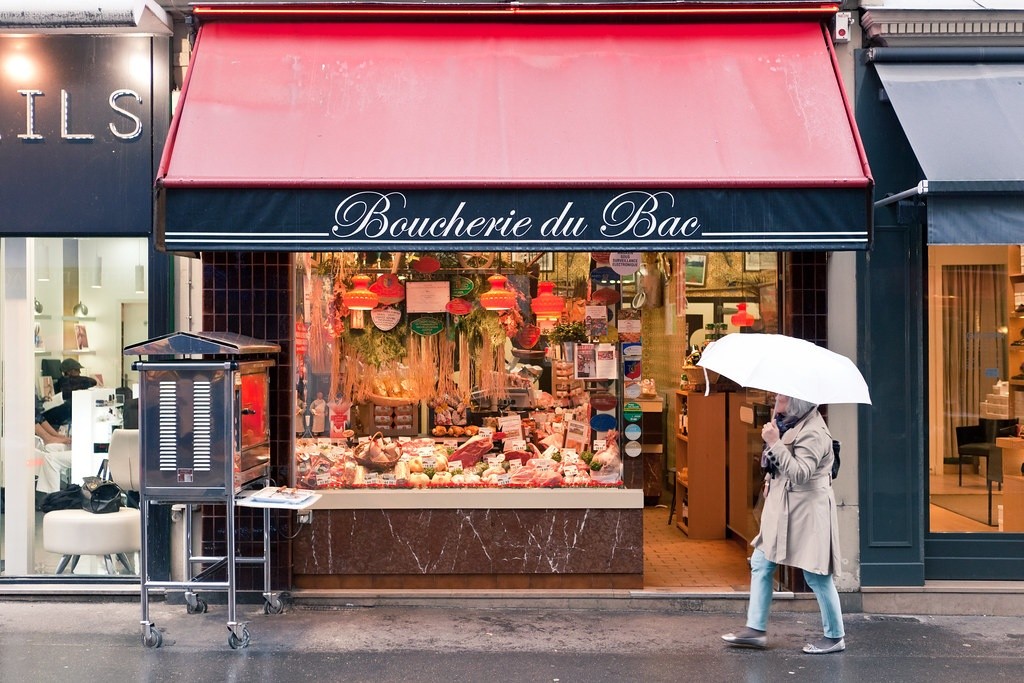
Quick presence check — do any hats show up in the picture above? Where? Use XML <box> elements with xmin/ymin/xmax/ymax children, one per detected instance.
<box><xmin>60</xmin><ymin>358</ymin><xmax>84</xmax><ymax>371</ymax></box>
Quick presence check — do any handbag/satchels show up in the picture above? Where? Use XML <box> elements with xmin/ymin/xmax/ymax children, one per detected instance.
<box><xmin>831</xmin><ymin>439</ymin><xmax>840</xmax><ymax>479</ymax></box>
<box><xmin>82</xmin><ymin>478</ymin><xmax>122</xmax><ymax>513</ymax></box>
<box><xmin>39</xmin><ymin>483</ymin><xmax>84</xmax><ymax>513</ymax></box>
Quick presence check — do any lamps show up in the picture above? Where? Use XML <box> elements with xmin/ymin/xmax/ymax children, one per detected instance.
<box><xmin>532</xmin><ymin>253</ymin><xmax>566</xmax><ymax>317</ymax></box>
<box><xmin>481</xmin><ymin>252</ymin><xmax>515</xmax><ymax>310</ymax></box>
<box><xmin>346</xmin><ymin>252</ymin><xmax>378</xmax><ymax>310</ymax></box>
<box><xmin>730</xmin><ymin>251</ymin><xmax>755</xmax><ymax>327</ymax></box>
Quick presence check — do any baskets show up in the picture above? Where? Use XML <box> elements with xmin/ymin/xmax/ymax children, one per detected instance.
<box><xmin>354</xmin><ymin>431</ymin><xmax>403</xmax><ymax>470</ymax></box>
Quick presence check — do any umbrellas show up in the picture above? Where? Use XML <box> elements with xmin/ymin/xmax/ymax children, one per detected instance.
<box><xmin>696</xmin><ymin>333</ymin><xmax>873</xmax><ymax>423</ymax></box>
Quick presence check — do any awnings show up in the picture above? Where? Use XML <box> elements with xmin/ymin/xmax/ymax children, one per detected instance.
<box><xmin>854</xmin><ymin>38</ymin><xmax>1024</xmax><ymax>244</ymax></box>
<box><xmin>150</xmin><ymin>14</ymin><xmax>875</xmax><ymax>254</ymax></box>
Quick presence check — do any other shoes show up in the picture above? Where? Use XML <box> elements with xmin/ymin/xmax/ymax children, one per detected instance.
<box><xmin>802</xmin><ymin>637</ymin><xmax>846</xmax><ymax>653</ymax></box>
<box><xmin>722</xmin><ymin>632</ymin><xmax>768</xmax><ymax>648</ymax></box>
<box><xmin>36</xmin><ymin>494</ymin><xmax>49</xmax><ymax>511</ymax></box>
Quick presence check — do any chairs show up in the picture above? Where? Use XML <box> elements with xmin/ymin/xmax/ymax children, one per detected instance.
<box><xmin>42</xmin><ymin>429</ymin><xmax>142</xmax><ymax>575</ymax></box>
<box><xmin>954</xmin><ymin>418</ymin><xmax>1019</xmax><ymax>485</ymax></box>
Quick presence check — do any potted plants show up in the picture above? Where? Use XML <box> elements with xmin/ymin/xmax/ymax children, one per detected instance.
<box><xmin>549</xmin><ymin>321</ymin><xmax>589</xmax><ymax>362</ymax></box>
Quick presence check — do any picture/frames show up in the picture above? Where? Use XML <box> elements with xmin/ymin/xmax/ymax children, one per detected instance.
<box><xmin>685</xmin><ymin>253</ymin><xmax>707</xmax><ymax>286</ymax></box>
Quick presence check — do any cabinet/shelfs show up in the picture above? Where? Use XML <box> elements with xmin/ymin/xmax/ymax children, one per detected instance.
<box><xmin>36</xmin><ymin>312</ymin><xmax>96</xmax><ymax>356</ymax></box>
<box><xmin>1007</xmin><ymin>314</ymin><xmax>1024</xmax><ymax>385</ymax></box>
<box><xmin>674</xmin><ymin>389</ymin><xmax>726</xmax><ymax>540</ymax></box>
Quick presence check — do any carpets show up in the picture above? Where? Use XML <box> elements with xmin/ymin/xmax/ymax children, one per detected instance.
<box><xmin>929</xmin><ymin>494</ymin><xmax>1003</xmax><ymax>526</ymax></box>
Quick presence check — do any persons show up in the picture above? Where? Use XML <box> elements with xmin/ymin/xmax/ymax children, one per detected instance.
<box><xmin>34</xmin><ymin>357</ymin><xmax>97</xmax><ymax>511</ymax></box>
<box><xmin>308</xmin><ymin>392</ymin><xmax>328</xmax><ymax>438</ymax></box>
<box><xmin>296</xmin><ymin>391</ymin><xmax>307</xmax><ymax>437</ymax></box>
<box><xmin>721</xmin><ymin>394</ymin><xmax>846</xmax><ymax>654</ymax></box>
